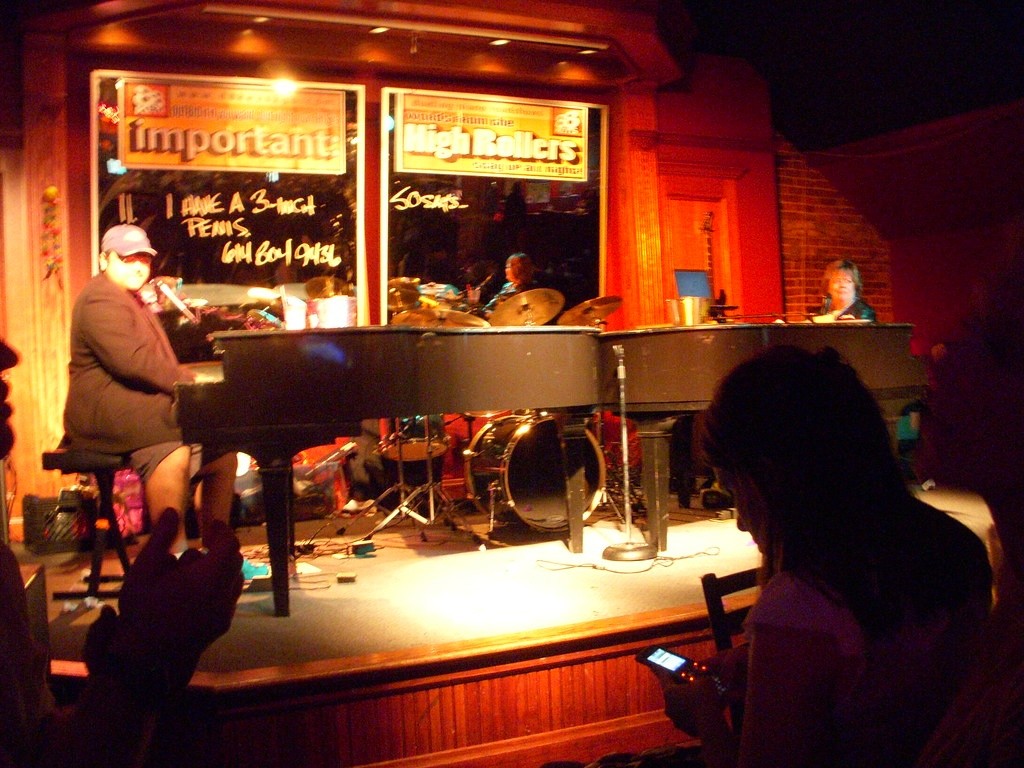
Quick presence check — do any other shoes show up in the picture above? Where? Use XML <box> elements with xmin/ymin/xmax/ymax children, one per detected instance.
<box><xmin>243</xmin><ymin>561</ymin><xmax>268</xmax><ymax>580</ymax></box>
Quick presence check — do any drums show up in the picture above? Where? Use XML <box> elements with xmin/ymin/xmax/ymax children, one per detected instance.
<box><xmin>377</xmin><ymin>439</ymin><xmax>447</xmax><ymax>507</ymax></box>
<box><xmin>388</xmin><ymin>277</ymin><xmax>421</xmax><ymax>308</ymax></box>
<box><xmin>463</xmin><ymin>415</ymin><xmax>606</xmax><ymax>532</ymax></box>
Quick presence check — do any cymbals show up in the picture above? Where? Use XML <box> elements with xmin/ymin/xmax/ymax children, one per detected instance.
<box><xmin>390</xmin><ymin>308</ymin><xmax>491</xmax><ymax>327</ymax></box>
<box><xmin>557</xmin><ymin>296</ymin><xmax>623</xmax><ymax>327</ymax></box>
<box><xmin>491</xmin><ymin>288</ymin><xmax>564</xmax><ymax>326</ymax></box>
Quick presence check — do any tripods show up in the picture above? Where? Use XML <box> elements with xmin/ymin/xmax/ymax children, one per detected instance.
<box><xmin>337</xmin><ymin>415</ymin><xmax>481</xmax><ymax>552</ymax></box>
<box><xmin>597</xmin><ymin>412</ymin><xmax>646</xmax><ymax>524</ymax></box>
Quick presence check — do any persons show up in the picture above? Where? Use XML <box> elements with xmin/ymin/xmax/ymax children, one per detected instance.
<box><xmin>1</xmin><ymin>335</ymin><xmax>246</xmax><ymax>768</ymax></box>
<box><xmin>63</xmin><ymin>222</ymin><xmax>273</xmax><ymax>579</ymax></box>
<box><xmin>646</xmin><ymin>340</ymin><xmax>994</xmax><ymax>768</ymax></box>
<box><xmin>906</xmin><ymin>242</ymin><xmax>1024</xmax><ymax>768</ymax></box>
<box><xmin>481</xmin><ymin>250</ymin><xmax>546</xmax><ymax>316</ymax></box>
<box><xmin>817</xmin><ymin>259</ymin><xmax>879</xmax><ymax>324</ymax></box>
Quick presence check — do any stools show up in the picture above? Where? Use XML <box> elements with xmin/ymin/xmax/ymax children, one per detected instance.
<box><xmin>42</xmin><ymin>451</ymin><xmax>132</xmax><ymax>600</ymax></box>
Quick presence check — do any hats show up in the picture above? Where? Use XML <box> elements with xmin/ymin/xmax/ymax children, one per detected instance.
<box><xmin>102</xmin><ymin>223</ymin><xmax>157</xmax><ymax>256</ymax></box>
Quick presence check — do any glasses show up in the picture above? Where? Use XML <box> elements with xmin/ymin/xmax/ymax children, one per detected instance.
<box><xmin>121</xmin><ymin>256</ymin><xmax>152</xmax><ymax>264</ymax></box>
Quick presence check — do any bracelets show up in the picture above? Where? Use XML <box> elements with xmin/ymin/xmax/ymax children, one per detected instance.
<box><xmin>81</xmin><ymin>605</ymin><xmax>204</xmax><ymax>706</ymax></box>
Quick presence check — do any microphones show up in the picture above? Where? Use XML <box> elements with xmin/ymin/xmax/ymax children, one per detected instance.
<box><xmin>821</xmin><ymin>293</ymin><xmax>832</xmax><ymax>315</ymax></box>
<box><xmin>157</xmin><ymin>281</ymin><xmax>198</xmax><ymax>324</ymax></box>
<box><xmin>475</xmin><ymin>273</ymin><xmax>496</xmax><ymax>291</ymax></box>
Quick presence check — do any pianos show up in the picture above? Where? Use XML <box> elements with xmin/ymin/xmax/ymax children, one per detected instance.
<box><xmin>598</xmin><ymin>321</ymin><xmax>930</xmax><ymax>553</ymax></box>
<box><xmin>173</xmin><ymin>327</ymin><xmax>604</xmax><ymax>617</ymax></box>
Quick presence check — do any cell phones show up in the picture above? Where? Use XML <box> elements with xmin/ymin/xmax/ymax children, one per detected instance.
<box><xmin>634</xmin><ymin>644</ymin><xmax>731</xmax><ymax>696</ymax></box>
